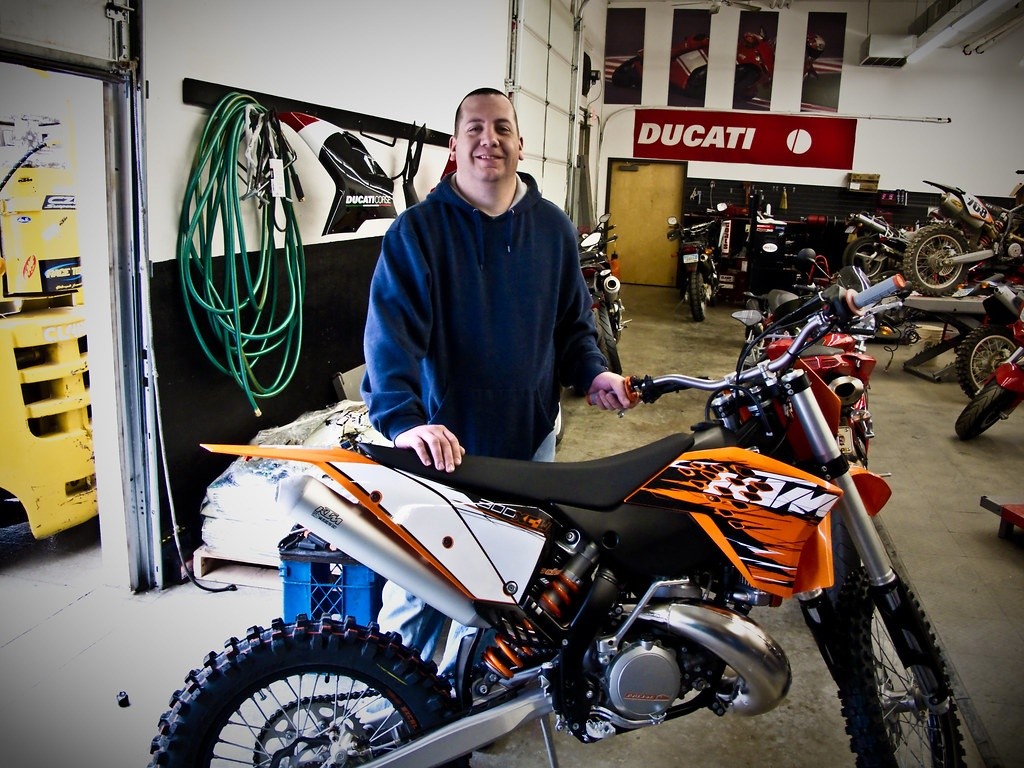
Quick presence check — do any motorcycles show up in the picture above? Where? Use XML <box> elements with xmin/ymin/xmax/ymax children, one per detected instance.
<box><xmin>841</xmin><ymin>209</ymin><xmax>906</xmax><ymax>282</ymax></box>
<box><xmin>577</xmin><ymin>212</ymin><xmax>634</xmax><ymax>383</ymax></box>
<box><xmin>147</xmin><ymin>272</ymin><xmax>966</xmax><ymax>768</ymax></box>
<box><xmin>903</xmin><ymin>169</ymin><xmax>1024</xmax><ymax>298</ymax></box>
<box><xmin>667</xmin><ymin>202</ymin><xmax>728</xmax><ymax>322</ymax></box>
<box><xmin>952</xmin><ymin>285</ymin><xmax>1023</xmax><ymax>443</ymax></box>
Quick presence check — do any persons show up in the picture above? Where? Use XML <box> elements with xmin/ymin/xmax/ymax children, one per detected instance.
<box><xmin>359</xmin><ymin>87</ymin><xmax>633</xmax><ymax>720</ymax></box>
